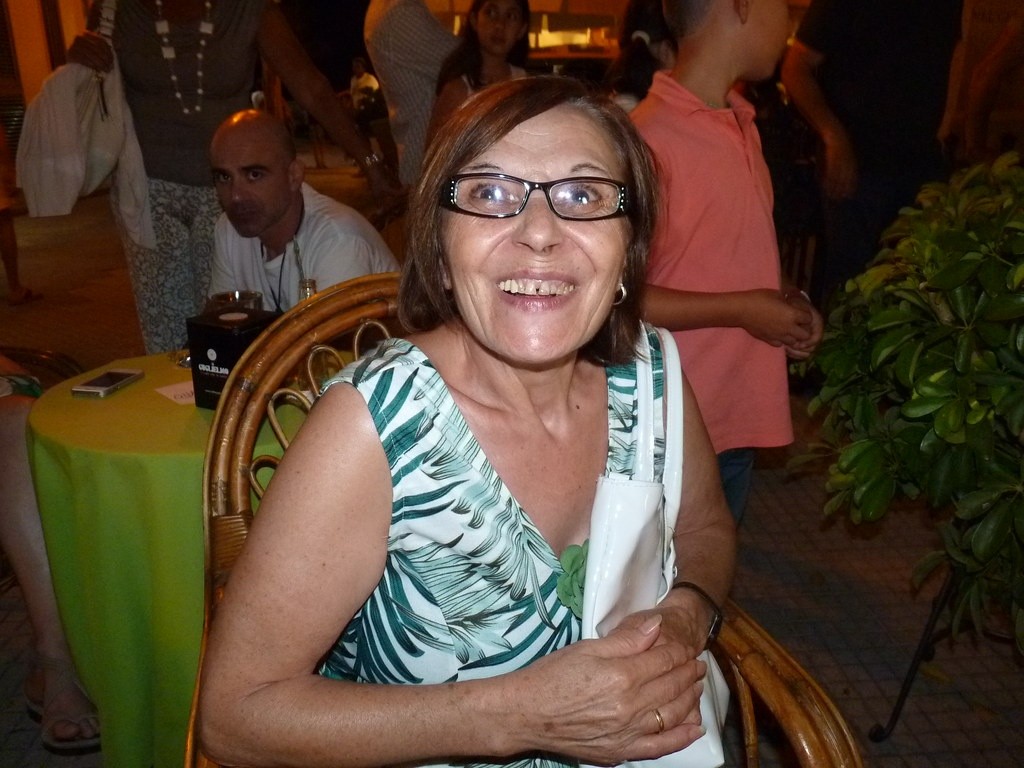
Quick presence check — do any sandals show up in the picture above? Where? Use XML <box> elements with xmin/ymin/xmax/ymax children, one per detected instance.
<box><xmin>26</xmin><ymin>656</ymin><xmax>102</xmax><ymax>756</ymax></box>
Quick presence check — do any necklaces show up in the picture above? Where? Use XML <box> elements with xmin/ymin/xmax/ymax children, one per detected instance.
<box><xmin>154</xmin><ymin>1</ymin><xmax>212</xmax><ymax>113</ymax></box>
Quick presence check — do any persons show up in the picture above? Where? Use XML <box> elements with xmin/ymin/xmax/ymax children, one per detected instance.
<box><xmin>68</xmin><ymin>0</ymin><xmax>1024</xmax><ymax>521</ymax></box>
<box><xmin>195</xmin><ymin>75</ymin><xmax>737</xmax><ymax>768</ymax></box>
<box><xmin>0</xmin><ymin>372</ymin><xmax>106</xmax><ymax>754</ymax></box>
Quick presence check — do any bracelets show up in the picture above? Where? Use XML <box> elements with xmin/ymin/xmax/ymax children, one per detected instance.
<box><xmin>786</xmin><ymin>290</ymin><xmax>813</xmax><ymax>300</ymax></box>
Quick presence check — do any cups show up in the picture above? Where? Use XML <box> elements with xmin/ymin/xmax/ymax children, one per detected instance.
<box><xmin>210</xmin><ymin>290</ymin><xmax>263</xmax><ymax>312</ymax></box>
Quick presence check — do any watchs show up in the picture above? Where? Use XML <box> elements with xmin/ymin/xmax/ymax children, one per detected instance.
<box><xmin>672</xmin><ymin>580</ymin><xmax>722</xmax><ymax>647</ymax></box>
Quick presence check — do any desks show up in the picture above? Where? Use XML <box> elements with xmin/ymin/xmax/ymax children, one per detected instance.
<box><xmin>24</xmin><ymin>350</ymin><xmax>354</xmax><ymax>768</ymax></box>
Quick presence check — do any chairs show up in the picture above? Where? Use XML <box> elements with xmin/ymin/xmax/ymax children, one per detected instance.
<box><xmin>184</xmin><ymin>273</ymin><xmax>864</xmax><ymax>768</ymax></box>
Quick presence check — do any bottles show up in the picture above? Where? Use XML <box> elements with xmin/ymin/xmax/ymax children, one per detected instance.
<box><xmin>294</xmin><ymin>279</ymin><xmax>330</xmax><ymax>392</ymax></box>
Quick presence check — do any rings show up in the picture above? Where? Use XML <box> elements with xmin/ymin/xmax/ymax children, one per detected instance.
<box><xmin>652</xmin><ymin>707</ymin><xmax>665</xmax><ymax>736</ymax></box>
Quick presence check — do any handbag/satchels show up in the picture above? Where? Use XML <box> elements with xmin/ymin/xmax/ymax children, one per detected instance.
<box><xmin>581</xmin><ymin>320</ymin><xmax>730</xmax><ymax>768</ymax></box>
<box><xmin>15</xmin><ymin>34</ymin><xmax>125</xmax><ymax>198</ymax></box>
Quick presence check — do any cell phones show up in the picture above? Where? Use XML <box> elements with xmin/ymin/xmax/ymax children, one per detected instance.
<box><xmin>71</xmin><ymin>369</ymin><xmax>143</xmax><ymax>398</ymax></box>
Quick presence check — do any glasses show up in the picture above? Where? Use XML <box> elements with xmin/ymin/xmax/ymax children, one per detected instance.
<box><xmin>438</xmin><ymin>174</ymin><xmax>636</xmax><ymax>222</ymax></box>
